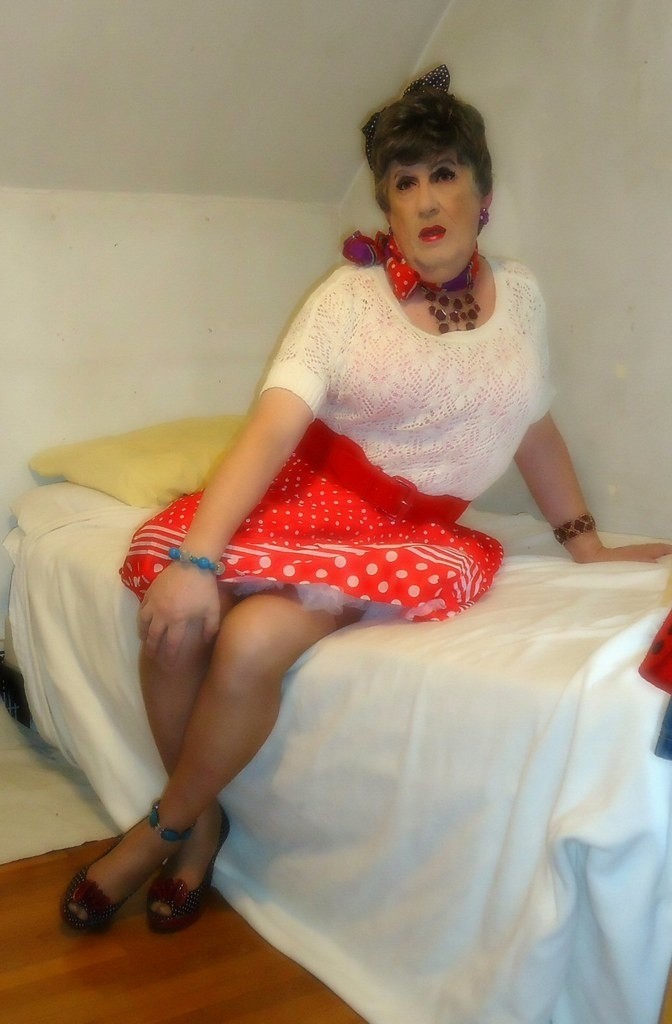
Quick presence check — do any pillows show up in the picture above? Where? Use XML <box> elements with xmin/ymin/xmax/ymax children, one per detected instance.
<box><xmin>29</xmin><ymin>413</ymin><xmax>247</xmax><ymax>509</ymax></box>
<box><xmin>7</xmin><ymin>483</ymin><xmax>131</xmax><ymax>542</ymax></box>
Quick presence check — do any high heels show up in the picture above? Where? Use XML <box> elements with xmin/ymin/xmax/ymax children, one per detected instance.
<box><xmin>60</xmin><ymin>815</ymin><xmax>163</xmax><ymax>932</ymax></box>
<box><xmin>146</xmin><ymin>802</ymin><xmax>230</xmax><ymax>933</ymax></box>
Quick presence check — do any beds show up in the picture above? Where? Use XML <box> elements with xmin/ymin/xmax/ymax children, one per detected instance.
<box><xmin>2</xmin><ymin>506</ymin><xmax>672</xmax><ymax>1024</ymax></box>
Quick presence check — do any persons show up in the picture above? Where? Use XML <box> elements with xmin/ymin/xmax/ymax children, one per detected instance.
<box><xmin>58</xmin><ymin>65</ymin><xmax>672</xmax><ymax>935</ymax></box>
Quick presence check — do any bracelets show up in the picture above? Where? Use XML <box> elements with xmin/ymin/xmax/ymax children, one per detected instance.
<box><xmin>554</xmin><ymin>511</ymin><xmax>595</xmax><ymax>546</ymax></box>
<box><xmin>167</xmin><ymin>546</ymin><xmax>226</xmax><ymax>577</ymax></box>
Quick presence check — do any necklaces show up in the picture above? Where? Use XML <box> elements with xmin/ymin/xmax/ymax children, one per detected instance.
<box><xmin>414</xmin><ymin>275</ymin><xmax>481</xmax><ymax>334</ymax></box>
